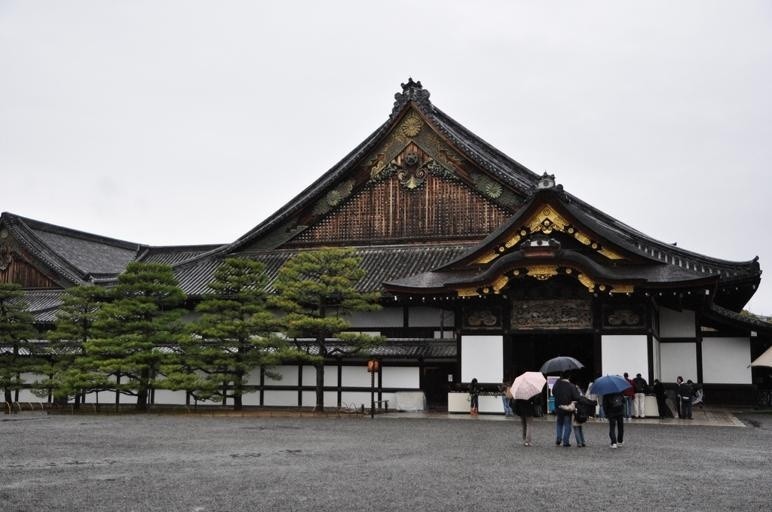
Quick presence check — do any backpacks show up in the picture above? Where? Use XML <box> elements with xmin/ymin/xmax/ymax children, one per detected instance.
<box><xmin>574</xmin><ymin>404</ymin><xmax>588</xmax><ymax>423</ymax></box>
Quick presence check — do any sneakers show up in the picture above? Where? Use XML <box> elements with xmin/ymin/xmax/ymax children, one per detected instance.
<box><xmin>610</xmin><ymin>442</ymin><xmax>623</xmax><ymax>448</ymax></box>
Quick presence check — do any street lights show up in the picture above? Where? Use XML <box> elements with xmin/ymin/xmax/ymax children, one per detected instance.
<box><xmin>367</xmin><ymin>359</ymin><xmax>380</xmax><ymax>419</ymax></box>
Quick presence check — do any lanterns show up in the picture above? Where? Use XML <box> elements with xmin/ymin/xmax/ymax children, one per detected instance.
<box><xmin>367</xmin><ymin>359</ymin><xmax>380</xmax><ymax>372</ymax></box>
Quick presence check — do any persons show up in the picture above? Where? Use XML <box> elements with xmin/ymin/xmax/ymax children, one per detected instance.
<box><xmin>468</xmin><ymin>354</ymin><xmax>701</xmax><ymax>450</ymax></box>
